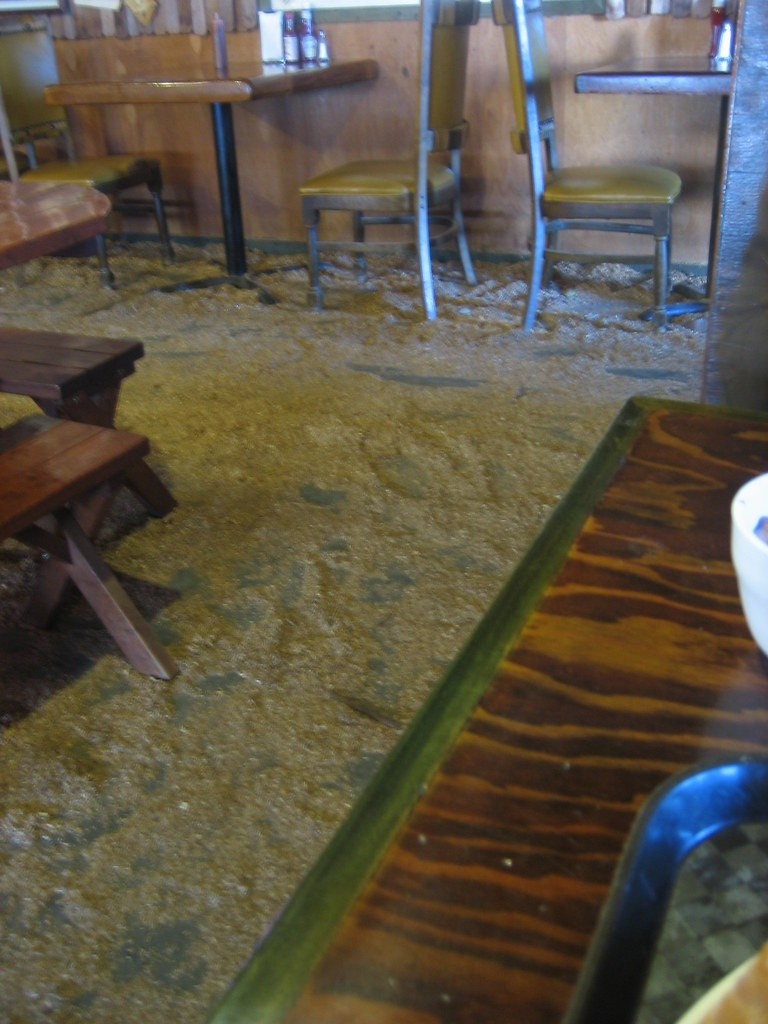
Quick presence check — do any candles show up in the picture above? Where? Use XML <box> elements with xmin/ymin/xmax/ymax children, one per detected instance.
<box><xmin>212</xmin><ymin>11</ymin><xmax>228</xmax><ymax>68</ymax></box>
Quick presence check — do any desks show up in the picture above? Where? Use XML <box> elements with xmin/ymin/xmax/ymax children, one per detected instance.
<box><xmin>42</xmin><ymin>59</ymin><xmax>377</xmax><ymax>307</ymax></box>
<box><xmin>575</xmin><ymin>53</ymin><xmax>733</xmax><ymax>323</ymax></box>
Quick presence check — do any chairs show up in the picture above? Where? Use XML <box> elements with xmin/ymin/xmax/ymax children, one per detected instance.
<box><xmin>0</xmin><ymin>70</ymin><xmax>175</xmax><ymax>295</ymax></box>
<box><xmin>298</xmin><ymin>0</ymin><xmax>480</xmax><ymax>319</ymax></box>
<box><xmin>491</xmin><ymin>0</ymin><xmax>681</xmax><ymax>333</ymax></box>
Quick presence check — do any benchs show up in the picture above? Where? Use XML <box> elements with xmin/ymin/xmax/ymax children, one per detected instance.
<box><xmin>0</xmin><ymin>412</ymin><xmax>180</xmax><ymax>680</ymax></box>
<box><xmin>0</xmin><ymin>322</ymin><xmax>177</xmax><ymax>520</ymax></box>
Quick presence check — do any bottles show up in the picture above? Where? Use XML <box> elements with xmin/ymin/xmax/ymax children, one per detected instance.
<box><xmin>259</xmin><ymin>1</ymin><xmax>331</xmax><ymax>68</ymax></box>
<box><xmin>48</xmin><ymin>1</ymin><xmax>258</xmax><ymax>39</ymax></box>
<box><xmin>709</xmin><ymin>1</ymin><xmax>734</xmax><ymax>72</ymax></box>
<box><xmin>212</xmin><ymin>12</ymin><xmax>228</xmax><ymax>69</ymax></box>
<box><xmin>606</xmin><ymin>0</ymin><xmax>711</xmax><ymax>19</ymax></box>
